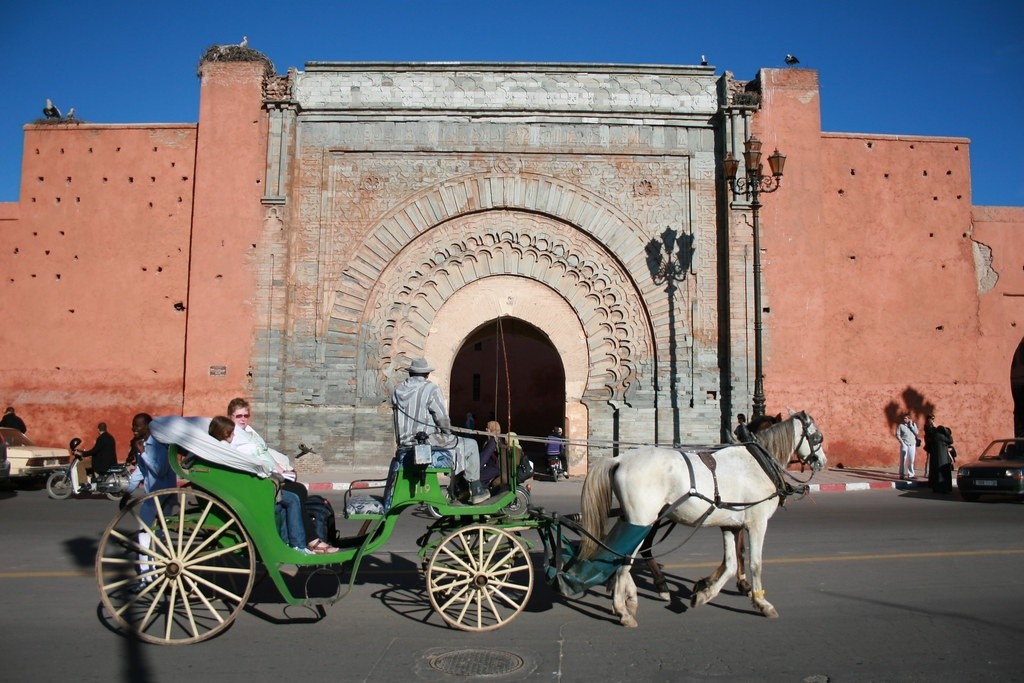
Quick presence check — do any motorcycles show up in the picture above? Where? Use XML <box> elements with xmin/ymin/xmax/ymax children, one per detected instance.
<box><xmin>46</xmin><ymin>451</ymin><xmax>132</xmax><ymax>501</ymax></box>
<box><xmin>547</xmin><ymin>454</ymin><xmax>564</xmax><ymax>484</ymax></box>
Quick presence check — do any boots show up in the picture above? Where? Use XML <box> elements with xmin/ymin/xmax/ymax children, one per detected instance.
<box><xmin>470</xmin><ymin>480</ymin><xmax>490</xmax><ymax>504</ymax></box>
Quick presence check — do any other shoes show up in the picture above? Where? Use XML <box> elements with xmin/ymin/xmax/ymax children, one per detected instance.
<box><xmin>78</xmin><ymin>484</ymin><xmax>91</xmax><ymax>491</ymax></box>
<box><xmin>909</xmin><ymin>476</ymin><xmax>917</xmax><ymax>479</ymax></box>
<box><xmin>900</xmin><ymin>476</ymin><xmax>904</xmax><ymax>480</ymax></box>
<box><xmin>563</xmin><ymin>472</ymin><xmax>569</xmax><ymax>479</ymax></box>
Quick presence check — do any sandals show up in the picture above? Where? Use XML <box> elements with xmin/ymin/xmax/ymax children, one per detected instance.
<box><xmin>308</xmin><ymin>540</ymin><xmax>339</xmax><ymax>554</ymax></box>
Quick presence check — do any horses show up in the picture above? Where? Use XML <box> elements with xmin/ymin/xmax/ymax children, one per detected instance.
<box><xmin>576</xmin><ymin>407</ymin><xmax>829</xmax><ymax>631</ymax></box>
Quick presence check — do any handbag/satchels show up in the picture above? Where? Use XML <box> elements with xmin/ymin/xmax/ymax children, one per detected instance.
<box><xmin>916</xmin><ymin>438</ymin><xmax>921</xmax><ymax>447</ymax></box>
<box><xmin>516</xmin><ymin>455</ymin><xmax>534</xmax><ymax>484</ymax></box>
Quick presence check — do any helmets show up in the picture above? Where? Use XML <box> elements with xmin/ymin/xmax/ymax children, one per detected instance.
<box><xmin>70</xmin><ymin>438</ymin><xmax>82</xmax><ymax>450</ymax></box>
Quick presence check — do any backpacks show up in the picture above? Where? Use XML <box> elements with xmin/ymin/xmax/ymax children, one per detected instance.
<box><xmin>303</xmin><ymin>495</ymin><xmax>335</xmax><ymax>546</ymax></box>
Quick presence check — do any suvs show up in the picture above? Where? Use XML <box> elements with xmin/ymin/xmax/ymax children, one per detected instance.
<box><xmin>0</xmin><ymin>430</ymin><xmax>11</xmax><ymax>488</ymax></box>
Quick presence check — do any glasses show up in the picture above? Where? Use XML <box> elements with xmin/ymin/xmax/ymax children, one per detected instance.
<box><xmin>232</xmin><ymin>414</ymin><xmax>250</xmax><ymax>418</ymax></box>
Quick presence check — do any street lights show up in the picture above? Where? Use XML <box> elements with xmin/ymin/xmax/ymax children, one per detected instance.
<box><xmin>724</xmin><ymin>135</ymin><xmax>787</xmax><ymax>420</ymax></box>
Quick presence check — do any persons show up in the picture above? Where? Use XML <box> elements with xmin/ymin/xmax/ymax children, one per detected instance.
<box><xmin>0</xmin><ymin>407</ymin><xmax>27</xmax><ymax>448</ymax></box>
<box><xmin>227</xmin><ymin>398</ymin><xmax>339</xmax><ymax>554</ymax></box>
<box><xmin>479</xmin><ymin>420</ymin><xmax>569</xmax><ymax>496</ymax></box>
<box><xmin>208</xmin><ymin>416</ymin><xmax>315</xmax><ymax>555</ymax></box>
<box><xmin>392</xmin><ymin>357</ymin><xmax>490</xmax><ymax>503</ymax></box>
<box><xmin>118</xmin><ymin>413</ymin><xmax>177</xmax><ymax>593</ymax></box>
<box><xmin>73</xmin><ymin>422</ymin><xmax>117</xmax><ymax>491</ymax></box>
<box><xmin>734</xmin><ymin>413</ymin><xmax>750</xmax><ymax>442</ymax></box>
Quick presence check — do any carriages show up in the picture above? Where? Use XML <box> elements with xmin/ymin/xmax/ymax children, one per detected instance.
<box><xmin>95</xmin><ymin>404</ymin><xmax>827</xmax><ymax>646</ymax></box>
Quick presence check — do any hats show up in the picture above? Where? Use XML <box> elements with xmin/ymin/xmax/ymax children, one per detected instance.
<box><xmin>404</xmin><ymin>358</ymin><xmax>434</xmax><ymax>373</ymax></box>
<box><xmin>4</xmin><ymin>407</ymin><xmax>14</xmax><ymax>413</ymax></box>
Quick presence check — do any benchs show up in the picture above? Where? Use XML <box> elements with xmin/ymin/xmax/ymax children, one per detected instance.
<box><xmin>392</xmin><ymin>404</ymin><xmax>453</xmax><ymax>476</ymax></box>
<box><xmin>343</xmin><ymin>452</ymin><xmax>402</xmax><ymax>520</ymax></box>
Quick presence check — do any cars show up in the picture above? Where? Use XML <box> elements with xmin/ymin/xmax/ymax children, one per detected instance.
<box><xmin>957</xmin><ymin>438</ymin><xmax>1024</xmax><ymax>503</ymax></box>
<box><xmin>0</xmin><ymin>427</ymin><xmax>71</xmax><ymax>490</ymax></box>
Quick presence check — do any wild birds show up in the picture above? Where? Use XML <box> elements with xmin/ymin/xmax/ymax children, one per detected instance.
<box><xmin>43</xmin><ymin>98</ymin><xmax>59</xmax><ymax>119</ymax></box>
<box><xmin>702</xmin><ymin>55</ymin><xmax>709</xmax><ymax>67</ymax></box>
<box><xmin>785</xmin><ymin>54</ymin><xmax>800</xmax><ymax>68</ymax></box>
<box><xmin>66</xmin><ymin>107</ymin><xmax>76</xmax><ymax>118</ymax></box>
<box><xmin>239</xmin><ymin>36</ymin><xmax>249</xmax><ymax>47</ymax></box>
